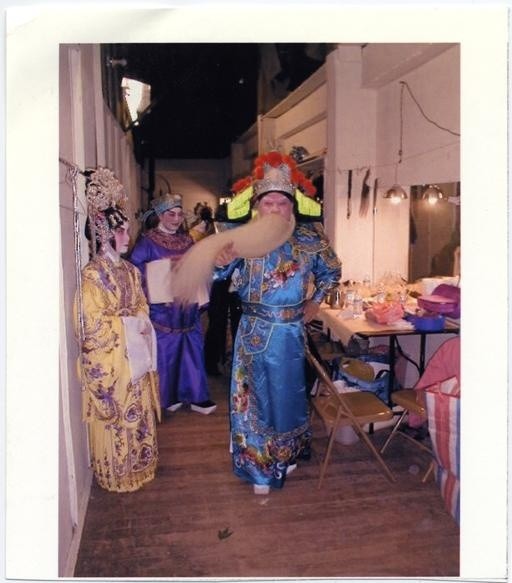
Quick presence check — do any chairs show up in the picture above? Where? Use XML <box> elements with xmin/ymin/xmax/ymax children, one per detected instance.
<box><xmin>189</xmin><ymin>401</ymin><xmax>220</xmax><ymax>415</ymax></box>
<box><xmin>252</xmin><ymin>482</ymin><xmax>270</xmax><ymax>495</ymax></box>
<box><xmin>165</xmin><ymin>402</ymin><xmax>183</xmax><ymax>415</ymax></box>
<box><xmin>283</xmin><ymin>463</ymin><xmax>298</xmax><ymax>473</ymax></box>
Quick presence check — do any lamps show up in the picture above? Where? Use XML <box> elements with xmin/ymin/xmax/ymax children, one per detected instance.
<box><xmin>303</xmin><ymin>344</ymin><xmax>439</xmax><ymax>490</ymax></box>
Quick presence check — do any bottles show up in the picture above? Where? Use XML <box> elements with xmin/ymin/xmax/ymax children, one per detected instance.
<box><xmin>254</xmin><ymin>166</ymin><xmax>294</xmax><ymax>198</ymax></box>
<box><xmin>150</xmin><ymin>193</ymin><xmax>183</xmax><ymax>216</ymax></box>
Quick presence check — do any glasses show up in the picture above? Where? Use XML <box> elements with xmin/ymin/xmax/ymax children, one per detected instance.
<box><xmin>422</xmin><ymin>184</ymin><xmax>442</xmax><ymax>204</ymax></box>
<box><xmin>383</xmin><ymin>162</ymin><xmax>407</xmax><ymax>204</ymax></box>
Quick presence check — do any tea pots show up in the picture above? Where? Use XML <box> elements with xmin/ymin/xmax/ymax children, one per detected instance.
<box><xmin>410</xmin><ymin>181</ymin><xmax>460</xmax><ymax>284</ymax></box>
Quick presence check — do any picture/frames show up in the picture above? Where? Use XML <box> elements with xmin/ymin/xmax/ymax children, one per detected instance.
<box><xmin>317</xmin><ymin>304</ymin><xmax>460</xmax><ymax>433</ymax></box>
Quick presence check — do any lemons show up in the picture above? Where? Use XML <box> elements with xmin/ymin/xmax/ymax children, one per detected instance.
<box><xmin>324</xmin><ymin>290</ymin><xmax>345</xmax><ymax>309</ymax></box>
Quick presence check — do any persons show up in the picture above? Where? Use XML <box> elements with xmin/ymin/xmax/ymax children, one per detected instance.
<box><xmin>188</xmin><ymin>206</ymin><xmax>217</xmax><ymax>242</ymax></box>
<box><xmin>128</xmin><ymin>191</ymin><xmax>217</xmax><ymax>414</ymax></box>
<box><xmin>169</xmin><ymin>150</ymin><xmax>342</xmax><ymax>494</ymax></box>
<box><xmin>73</xmin><ymin>167</ymin><xmax>162</xmax><ymax>493</ymax></box>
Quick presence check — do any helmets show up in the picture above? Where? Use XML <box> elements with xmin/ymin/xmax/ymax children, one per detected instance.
<box><xmin>415</xmin><ymin>315</ymin><xmax>444</xmax><ymax>331</ymax></box>
